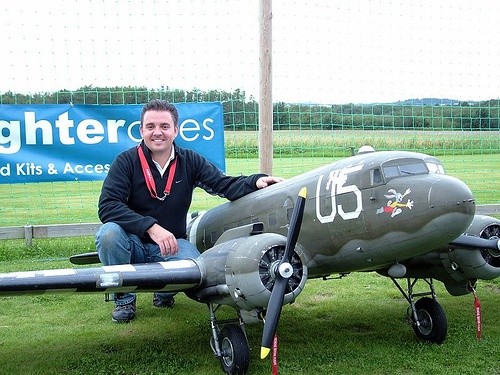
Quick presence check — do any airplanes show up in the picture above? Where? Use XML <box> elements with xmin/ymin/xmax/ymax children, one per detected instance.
<box><xmin>1</xmin><ymin>145</ymin><xmax>500</xmax><ymax>375</ymax></box>
